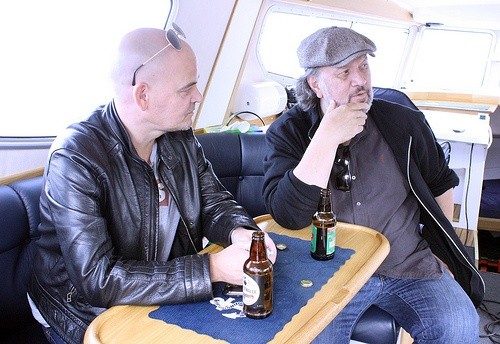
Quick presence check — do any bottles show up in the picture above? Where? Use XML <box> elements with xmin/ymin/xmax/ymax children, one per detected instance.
<box><xmin>311</xmin><ymin>188</ymin><xmax>337</xmax><ymax>262</ymax></box>
<box><xmin>242</xmin><ymin>231</ymin><xmax>273</xmax><ymax>318</ymax></box>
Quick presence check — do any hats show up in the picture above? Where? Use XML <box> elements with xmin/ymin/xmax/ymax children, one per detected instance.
<box><xmin>297</xmin><ymin>25</ymin><xmax>377</xmax><ymax>69</ymax></box>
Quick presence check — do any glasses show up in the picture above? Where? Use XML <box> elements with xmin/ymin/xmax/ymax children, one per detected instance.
<box><xmin>131</xmin><ymin>22</ymin><xmax>187</xmax><ymax>86</ymax></box>
<box><xmin>331</xmin><ymin>144</ymin><xmax>352</xmax><ymax>192</ymax></box>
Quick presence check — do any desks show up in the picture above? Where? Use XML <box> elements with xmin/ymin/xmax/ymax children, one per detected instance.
<box><xmin>84</xmin><ymin>214</ymin><xmax>390</xmax><ymax>344</ymax></box>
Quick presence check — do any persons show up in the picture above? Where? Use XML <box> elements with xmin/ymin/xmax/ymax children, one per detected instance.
<box><xmin>25</xmin><ymin>22</ymin><xmax>277</xmax><ymax>344</ymax></box>
<box><xmin>261</xmin><ymin>26</ymin><xmax>485</xmax><ymax>344</ymax></box>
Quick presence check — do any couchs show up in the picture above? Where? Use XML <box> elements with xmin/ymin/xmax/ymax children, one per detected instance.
<box><xmin>0</xmin><ymin>132</ymin><xmax>400</xmax><ymax>344</ymax></box>
